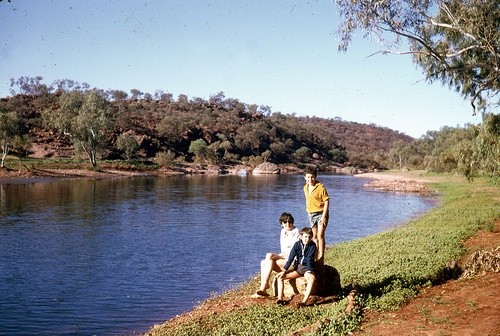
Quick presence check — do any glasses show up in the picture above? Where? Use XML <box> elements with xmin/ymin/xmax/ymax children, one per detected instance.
<box><xmin>284</xmin><ymin>221</ymin><xmax>292</xmax><ymax>223</ymax></box>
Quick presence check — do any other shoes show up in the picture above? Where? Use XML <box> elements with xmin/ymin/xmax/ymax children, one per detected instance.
<box><xmin>296</xmin><ymin>301</ymin><xmax>305</xmax><ymax>308</ymax></box>
<box><xmin>276</xmin><ymin>300</ymin><xmax>287</xmax><ymax>305</ymax></box>
<box><xmin>251</xmin><ymin>290</ymin><xmax>270</xmax><ymax>298</ymax></box>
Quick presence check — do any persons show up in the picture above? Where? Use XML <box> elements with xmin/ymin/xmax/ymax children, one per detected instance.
<box><xmin>272</xmin><ymin>226</ymin><xmax>320</xmax><ymax>308</ymax></box>
<box><xmin>303</xmin><ymin>164</ymin><xmax>331</xmax><ymax>264</ymax></box>
<box><xmin>250</xmin><ymin>212</ymin><xmax>302</xmax><ymax>300</ymax></box>
